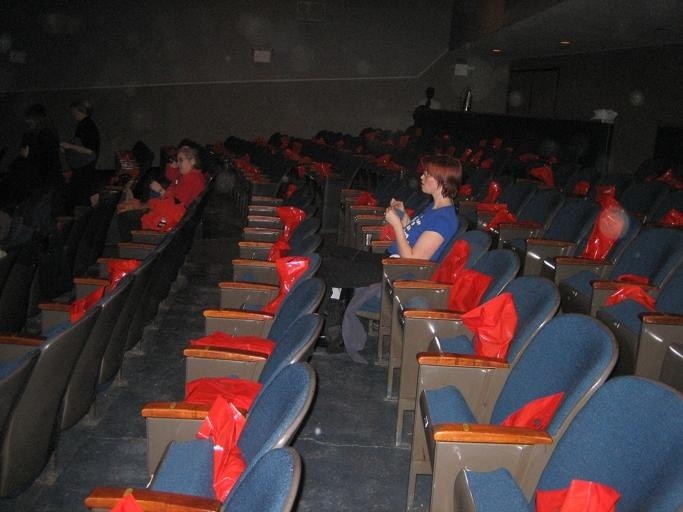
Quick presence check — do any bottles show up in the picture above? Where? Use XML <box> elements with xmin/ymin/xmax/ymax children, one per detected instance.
<box><xmin>461</xmin><ymin>87</ymin><xmax>470</xmax><ymax>112</ymax></box>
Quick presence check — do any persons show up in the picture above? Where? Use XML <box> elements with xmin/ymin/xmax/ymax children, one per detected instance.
<box><xmin>312</xmin><ymin>152</ymin><xmax>463</xmax><ymax>355</ymax></box>
<box><xmin>2</xmin><ymin>96</ymin><xmax>210</xmax><ymax>304</ymax></box>
